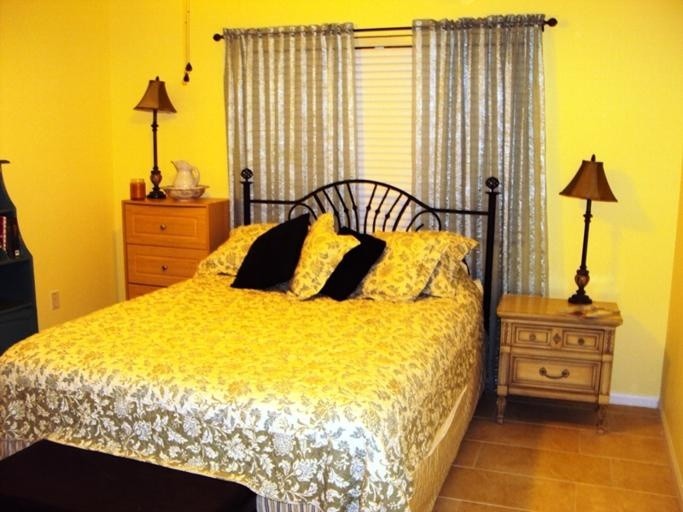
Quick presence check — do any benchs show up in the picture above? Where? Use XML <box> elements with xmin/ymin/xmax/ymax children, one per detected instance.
<box><xmin>0</xmin><ymin>439</ymin><xmax>257</xmax><ymax>512</ymax></box>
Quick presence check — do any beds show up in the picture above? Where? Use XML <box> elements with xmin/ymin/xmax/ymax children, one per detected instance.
<box><xmin>0</xmin><ymin>169</ymin><xmax>501</xmax><ymax>512</ymax></box>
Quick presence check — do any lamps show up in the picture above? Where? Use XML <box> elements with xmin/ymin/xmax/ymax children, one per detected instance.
<box><xmin>559</xmin><ymin>154</ymin><xmax>618</xmax><ymax>305</ymax></box>
<box><xmin>133</xmin><ymin>75</ymin><xmax>177</xmax><ymax>199</ymax></box>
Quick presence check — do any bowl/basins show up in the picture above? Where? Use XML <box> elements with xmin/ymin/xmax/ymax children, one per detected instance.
<box><xmin>158</xmin><ymin>184</ymin><xmax>209</xmax><ymax>201</ymax></box>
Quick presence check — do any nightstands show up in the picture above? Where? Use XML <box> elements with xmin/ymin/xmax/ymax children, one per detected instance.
<box><xmin>122</xmin><ymin>196</ymin><xmax>230</xmax><ymax>301</ymax></box>
<box><xmin>496</xmin><ymin>294</ymin><xmax>623</xmax><ymax>434</ymax></box>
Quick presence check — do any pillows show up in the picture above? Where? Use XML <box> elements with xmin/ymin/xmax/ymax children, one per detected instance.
<box><xmin>276</xmin><ymin>210</ymin><xmax>362</xmax><ymax>301</ymax></box>
<box><xmin>230</xmin><ymin>212</ymin><xmax>310</xmax><ymax>289</ymax></box>
<box><xmin>354</xmin><ymin>230</ymin><xmax>450</xmax><ymax>304</ymax></box>
<box><xmin>319</xmin><ymin>226</ymin><xmax>386</xmax><ymax>301</ymax></box>
<box><xmin>418</xmin><ymin>229</ymin><xmax>480</xmax><ymax>300</ymax></box>
<box><xmin>192</xmin><ymin>222</ymin><xmax>280</xmax><ymax>276</ymax></box>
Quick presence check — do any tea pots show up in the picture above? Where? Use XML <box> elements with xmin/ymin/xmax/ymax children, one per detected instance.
<box><xmin>170</xmin><ymin>160</ymin><xmax>200</xmax><ymax>187</ymax></box>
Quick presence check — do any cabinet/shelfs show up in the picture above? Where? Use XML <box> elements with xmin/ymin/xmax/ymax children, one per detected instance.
<box><xmin>0</xmin><ymin>159</ymin><xmax>39</xmax><ymax>355</ymax></box>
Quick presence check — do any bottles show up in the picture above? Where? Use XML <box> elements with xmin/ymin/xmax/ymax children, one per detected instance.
<box><xmin>129</xmin><ymin>178</ymin><xmax>146</xmax><ymax>201</ymax></box>
